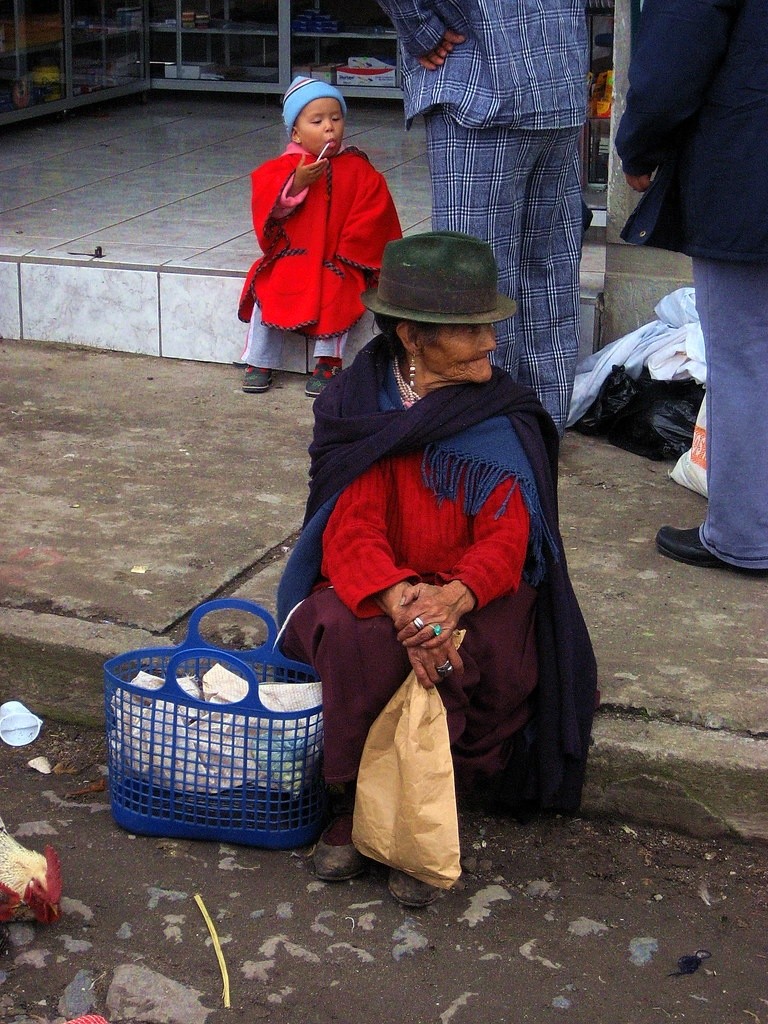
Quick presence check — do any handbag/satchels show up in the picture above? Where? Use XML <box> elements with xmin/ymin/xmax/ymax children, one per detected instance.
<box><xmin>351</xmin><ymin>629</ymin><xmax>463</xmax><ymax>890</ymax></box>
<box><xmin>668</xmin><ymin>394</ymin><xmax>710</xmax><ymax>498</ymax></box>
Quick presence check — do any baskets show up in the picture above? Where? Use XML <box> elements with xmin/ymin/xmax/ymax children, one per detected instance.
<box><xmin>103</xmin><ymin>599</ymin><xmax>328</xmax><ymax>848</ymax></box>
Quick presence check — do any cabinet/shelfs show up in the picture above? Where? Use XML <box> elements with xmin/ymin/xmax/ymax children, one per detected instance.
<box><xmin>0</xmin><ymin>0</ymin><xmax>150</xmax><ymax>127</ymax></box>
<box><xmin>150</xmin><ymin>0</ymin><xmax>403</xmax><ymax>108</ymax></box>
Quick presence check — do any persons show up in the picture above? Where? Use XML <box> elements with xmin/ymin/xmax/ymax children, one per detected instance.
<box><xmin>276</xmin><ymin>233</ymin><xmax>599</xmax><ymax>910</ymax></box>
<box><xmin>236</xmin><ymin>79</ymin><xmax>402</xmax><ymax>400</ymax></box>
<box><xmin>614</xmin><ymin>1</ymin><xmax>767</xmax><ymax>576</ymax></box>
<box><xmin>378</xmin><ymin>0</ymin><xmax>594</xmax><ymax>444</ymax></box>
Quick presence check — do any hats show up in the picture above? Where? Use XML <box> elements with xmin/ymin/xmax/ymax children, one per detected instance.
<box><xmin>360</xmin><ymin>231</ymin><xmax>517</xmax><ymax>323</ymax></box>
<box><xmin>283</xmin><ymin>76</ymin><xmax>347</xmax><ymax>143</ymax></box>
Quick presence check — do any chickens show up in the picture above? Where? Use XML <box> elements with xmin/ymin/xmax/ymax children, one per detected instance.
<box><xmin>0</xmin><ymin>815</ymin><xmax>62</xmax><ymax>924</ymax></box>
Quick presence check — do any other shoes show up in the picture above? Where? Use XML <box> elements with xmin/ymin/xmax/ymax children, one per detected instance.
<box><xmin>312</xmin><ymin>813</ymin><xmax>365</xmax><ymax>881</ymax></box>
<box><xmin>388</xmin><ymin>868</ymin><xmax>440</xmax><ymax>906</ymax></box>
<box><xmin>305</xmin><ymin>363</ymin><xmax>343</xmax><ymax>398</ymax></box>
<box><xmin>242</xmin><ymin>366</ymin><xmax>273</xmax><ymax>392</ymax></box>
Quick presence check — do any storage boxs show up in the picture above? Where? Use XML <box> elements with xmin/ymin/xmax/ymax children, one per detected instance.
<box><xmin>292</xmin><ymin>62</ymin><xmax>344</xmax><ymax>87</ymax></box>
<box><xmin>336</xmin><ymin>57</ymin><xmax>397</xmax><ymax>89</ymax></box>
<box><xmin>164</xmin><ymin>61</ymin><xmax>214</xmax><ymax>80</ymax></box>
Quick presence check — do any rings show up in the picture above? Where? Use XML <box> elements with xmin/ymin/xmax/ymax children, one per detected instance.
<box><xmin>414</xmin><ymin>618</ymin><xmax>424</xmax><ymax>630</ymax></box>
<box><xmin>429</xmin><ymin>624</ymin><xmax>442</xmax><ymax>636</ymax></box>
<box><xmin>436</xmin><ymin>660</ymin><xmax>454</xmax><ymax>677</ymax></box>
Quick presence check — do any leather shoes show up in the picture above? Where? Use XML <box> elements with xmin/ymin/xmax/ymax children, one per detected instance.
<box><xmin>655</xmin><ymin>526</ymin><xmax>767</xmax><ymax>578</ymax></box>
